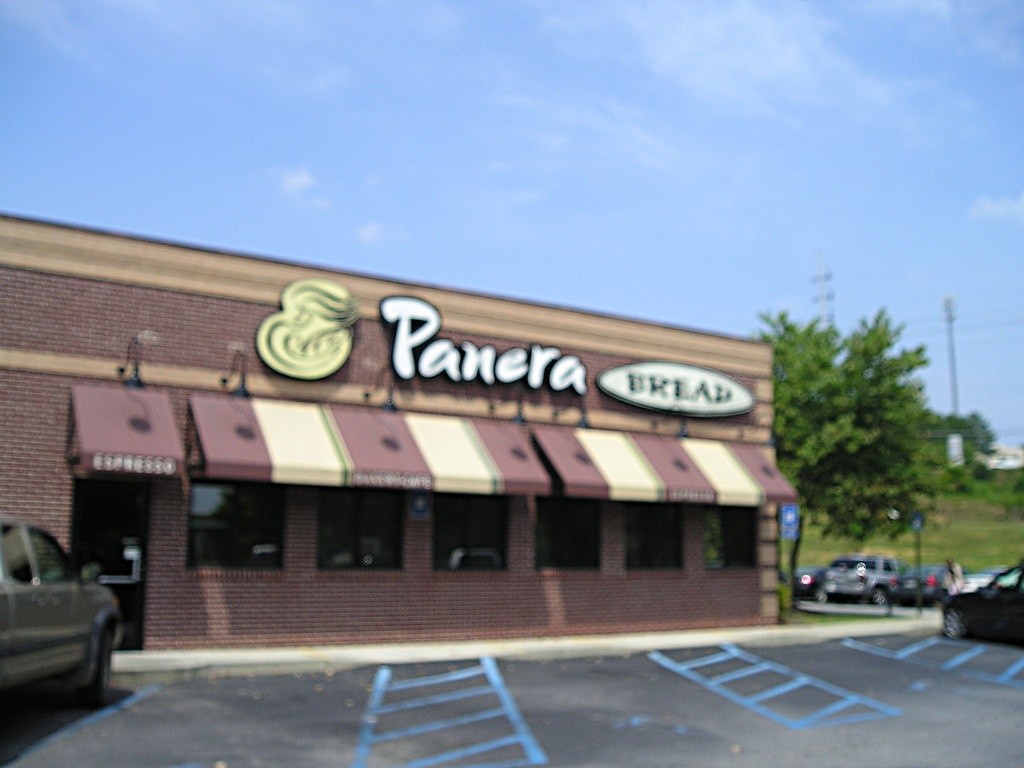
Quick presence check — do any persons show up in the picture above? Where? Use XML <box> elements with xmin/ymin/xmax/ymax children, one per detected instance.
<box><xmin>941</xmin><ymin>559</ymin><xmax>962</xmax><ymax>596</ymax></box>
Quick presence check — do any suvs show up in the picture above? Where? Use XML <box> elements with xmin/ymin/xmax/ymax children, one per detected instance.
<box><xmin>825</xmin><ymin>553</ymin><xmax>910</xmax><ymax>606</ymax></box>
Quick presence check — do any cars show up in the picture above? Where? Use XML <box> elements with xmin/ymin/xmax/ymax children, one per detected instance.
<box><xmin>942</xmin><ymin>559</ymin><xmax>1024</xmax><ymax>646</ymax></box>
<box><xmin>1</xmin><ymin>519</ymin><xmax>124</xmax><ymax>709</ymax></box>
<box><xmin>946</xmin><ymin>566</ymin><xmax>1006</xmax><ymax>607</ymax></box>
<box><xmin>888</xmin><ymin>560</ymin><xmax>966</xmax><ymax>607</ymax></box>
<box><xmin>793</xmin><ymin>567</ymin><xmax>828</xmax><ymax>602</ymax></box>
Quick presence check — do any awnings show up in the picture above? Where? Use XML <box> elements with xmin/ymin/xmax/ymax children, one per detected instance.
<box><xmin>71</xmin><ymin>387</ymin><xmax>185</xmax><ymax>481</ymax></box>
<box><xmin>188</xmin><ymin>392</ymin><xmax>552</xmax><ymax>495</ymax></box>
<box><xmin>530</xmin><ymin>423</ymin><xmax>800</xmax><ymax>507</ymax></box>
